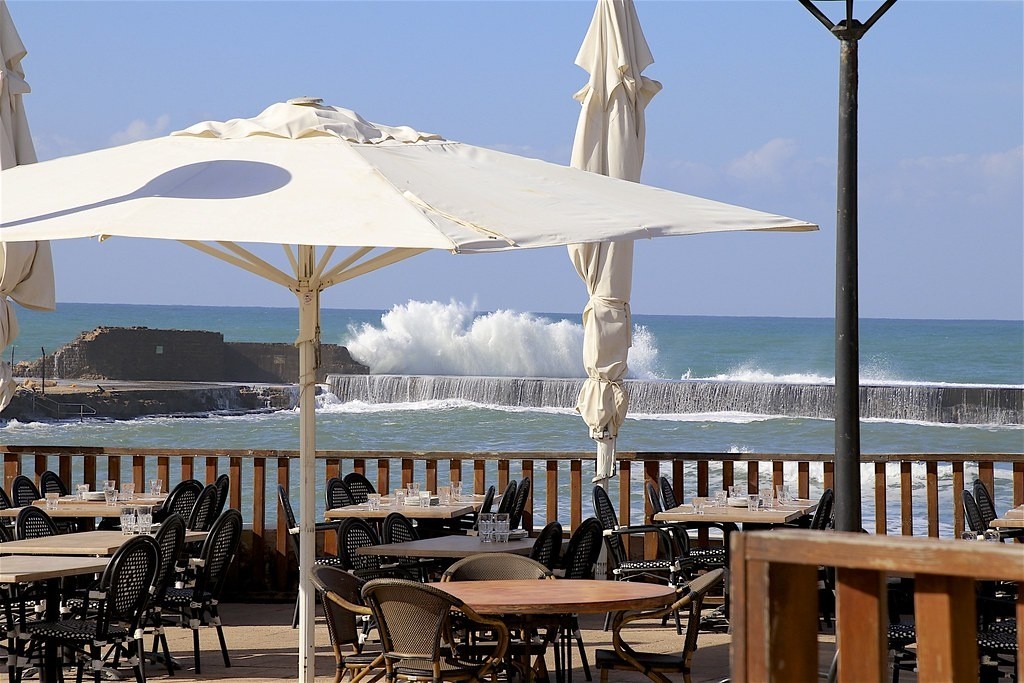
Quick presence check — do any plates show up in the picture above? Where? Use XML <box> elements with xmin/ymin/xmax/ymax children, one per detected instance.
<box><xmin>83</xmin><ymin>492</ymin><xmax>105</xmax><ymax>500</ymax></box>
<box><xmin>404</xmin><ymin>497</ymin><xmax>419</xmax><ymax>505</ymax></box>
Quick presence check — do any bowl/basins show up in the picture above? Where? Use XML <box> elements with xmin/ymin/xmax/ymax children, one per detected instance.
<box><xmin>45</xmin><ymin>493</ymin><xmax>59</xmax><ymax>499</ymax></box>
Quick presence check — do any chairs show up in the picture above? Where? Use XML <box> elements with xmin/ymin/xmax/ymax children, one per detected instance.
<box><xmin>0</xmin><ymin>471</ymin><xmax>1024</xmax><ymax>683</ymax></box>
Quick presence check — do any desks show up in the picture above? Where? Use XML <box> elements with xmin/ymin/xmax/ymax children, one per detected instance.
<box><xmin>324</xmin><ymin>505</ymin><xmax>473</xmax><ymax>567</ymax></box>
<box><xmin>395</xmin><ymin>580</ymin><xmax>677</xmax><ymax>683</ymax></box>
<box><xmin>654</xmin><ymin>507</ymin><xmax>801</xmax><ymax>634</ymax></box>
<box><xmin>694</xmin><ymin>497</ymin><xmax>820</xmax><ymax>566</ymax></box>
<box><xmin>379</xmin><ymin>493</ymin><xmax>502</xmax><ymax>536</ymax></box>
<box><xmin>0</xmin><ymin>504</ymin><xmax>155</xmax><ymax>609</ymax></box>
<box><xmin>0</xmin><ymin>530</ymin><xmax>211</xmax><ymax>667</ymax></box>
<box><xmin>356</xmin><ymin>535</ymin><xmax>570</xmax><ymax>663</ymax></box>
<box><xmin>990</xmin><ymin>503</ymin><xmax>1024</xmax><ymax>543</ymax></box>
<box><xmin>61</xmin><ymin>492</ymin><xmax>170</xmax><ymax>581</ymax></box>
<box><xmin>0</xmin><ymin>555</ymin><xmax>110</xmax><ymax>683</ymax></box>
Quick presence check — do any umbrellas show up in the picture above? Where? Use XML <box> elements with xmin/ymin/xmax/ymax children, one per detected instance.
<box><xmin>567</xmin><ymin>0</ymin><xmax>663</xmax><ymax>580</ymax></box>
<box><xmin>0</xmin><ymin>97</ymin><xmax>819</xmax><ymax>683</ymax></box>
<box><xmin>0</xmin><ymin>0</ymin><xmax>56</xmax><ymax>493</ymax></box>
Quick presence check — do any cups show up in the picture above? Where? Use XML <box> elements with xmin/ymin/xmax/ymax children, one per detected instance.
<box><xmin>368</xmin><ymin>494</ymin><xmax>381</xmax><ymax>512</ymax></box>
<box><xmin>76</xmin><ymin>483</ymin><xmax>89</xmax><ymax>501</ymax></box>
<box><xmin>120</xmin><ymin>505</ymin><xmax>152</xmax><ymax>535</ymax></box>
<box><xmin>437</xmin><ymin>482</ymin><xmax>462</xmax><ymax>507</ymax></box>
<box><xmin>149</xmin><ymin>479</ymin><xmax>163</xmax><ymax>496</ymax></box>
<box><xmin>46</xmin><ymin>497</ymin><xmax>58</xmax><ymax>510</ymax></box>
<box><xmin>394</xmin><ymin>489</ymin><xmax>407</xmax><ymax>507</ymax></box>
<box><xmin>407</xmin><ymin>483</ymin><xmax>419</xmax><ymax>496</ymax></box>
<box><xmin>430</xmin><ymin>498</ymin><xmax>438</xmax><ymax>506</ymax></box>
<box><xmin>479</xmin><ymin>513</ymin><xmax>509</xmax><ymax>542</ymax></box>
<box><xmin>692</xmin><ymin>482</ymin><xmax>789</xmax><ymax>514</ymax></box>
<box><xmin>419</xmin><ymin>491</ymin><xmax>431</xmax><ymax>507</ymax></box>
<box><xmin>105</xmin><ymin>490</ymin><xmax>118</xmax><ymax>507</ymax></box>
<box><xmin>122</xmin><ymin>483</ymin><xmax>135</xmax><ymax>501</ymax></box>
<box><xmin>104</xmin><ymin>480</ymin><xmax>115</xmax><ymax>491</ymax></box>
